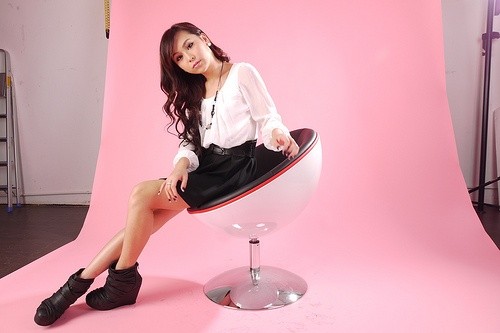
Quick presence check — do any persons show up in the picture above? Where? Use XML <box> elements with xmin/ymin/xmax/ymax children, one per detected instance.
<box><xmin>32</xmin><ymin>21</ymin><xmax>301</xmax><ymax>328</ymax></box>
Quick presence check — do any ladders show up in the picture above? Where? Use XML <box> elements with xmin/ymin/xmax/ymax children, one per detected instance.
<box><xmin>0</xmin><ymin>48</ymin><xmax>21</xmax><ymax>214</ymax></box>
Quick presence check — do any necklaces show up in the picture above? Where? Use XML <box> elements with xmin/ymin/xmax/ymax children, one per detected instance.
<box><xmin>198</xmin><ymin>60</ymin><xmax>225</xmax><ymax>130</ymax></box>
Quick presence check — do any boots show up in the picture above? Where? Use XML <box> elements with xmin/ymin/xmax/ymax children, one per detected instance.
<box><xmin>34</xmin><ymin>267</ymin><xmax>94</xmax><ymax>327</ymax></box>
<box><xmin>86</xmin><ymin>260</ymin><xmax>142</xmax><ymax>311</ymax></box>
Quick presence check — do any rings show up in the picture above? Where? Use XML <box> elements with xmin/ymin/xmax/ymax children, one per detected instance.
<box><xmin>166</xmin><ymin>183</ymin><xmax>172</xmax><ymax>186</ymax></box>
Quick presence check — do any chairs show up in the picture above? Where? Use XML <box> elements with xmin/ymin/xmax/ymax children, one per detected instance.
<box><xmin>186</xmin><ymin>126</ymin><xmax>323</xmax><ymax>311</ymax></box>
